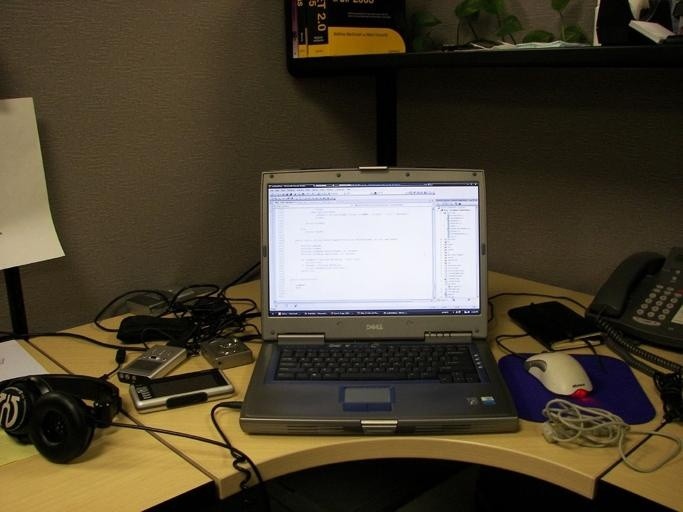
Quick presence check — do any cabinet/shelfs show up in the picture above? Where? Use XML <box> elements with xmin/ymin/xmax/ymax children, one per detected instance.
<box><xmin>282</xmin><ymin>3</ymin><xmax>683</xmax><ymax>100</ymax></box>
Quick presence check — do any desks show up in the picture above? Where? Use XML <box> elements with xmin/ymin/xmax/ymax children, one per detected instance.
<box><xmin>0</xmin><ymin>270</ymin><xmax>683</xmax><ymax>512</ymax></box>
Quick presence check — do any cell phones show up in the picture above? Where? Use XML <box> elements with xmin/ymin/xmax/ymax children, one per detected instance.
<box><xmin>117</xmin><ymin>345</ymin><xmax>186</xmax><ymax>384</ymax></box>
<box><xmin>129</xmin><ymin>368</ymin><xmax>235</xmax><ymax>414</ymax></box>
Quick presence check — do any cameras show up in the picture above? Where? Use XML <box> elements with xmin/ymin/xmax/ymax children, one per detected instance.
<box><xmin>199</xmin><ymin>336</ymin><xmax>254</xmax><ymax>370</ymax></box>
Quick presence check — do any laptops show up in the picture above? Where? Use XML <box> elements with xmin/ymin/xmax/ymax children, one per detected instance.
<box><xmin>239</xmin><ymin>166</ymin><xmax>520</xmax><ymax>436</ymax></box>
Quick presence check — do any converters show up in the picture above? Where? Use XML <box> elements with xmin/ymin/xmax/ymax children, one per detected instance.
<box><xmin>116</xmin><ymin>315</ymin><xmax>194</xmax><ymax>344</ymax></box>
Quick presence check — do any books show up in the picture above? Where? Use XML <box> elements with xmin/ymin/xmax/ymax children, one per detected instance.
<box><xmin>508</xmin><ymin>301</ymin><xmax>603</xmax><ymax>350</ymax></box>
<box><xmin>291</xmin><ymin>0</ymin><xmax>405</xmax><ymax>59</ymax></box>
<box><xmin>628</xmin><ymin>20</ymin><xmax>676</xmax><ymax>43</ymax></box>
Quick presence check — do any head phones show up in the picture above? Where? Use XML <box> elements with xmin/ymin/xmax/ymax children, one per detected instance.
<box><xmin>0</xmin><ymin>373</ymin><xmax>123</xmax><ymax>465</ymax></box>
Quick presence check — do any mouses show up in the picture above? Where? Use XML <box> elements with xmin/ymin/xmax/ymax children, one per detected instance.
<box><xmin>523</xmin><ymin>351</ymin><xmax>593</xmax><ymax>399</ymax></box>
